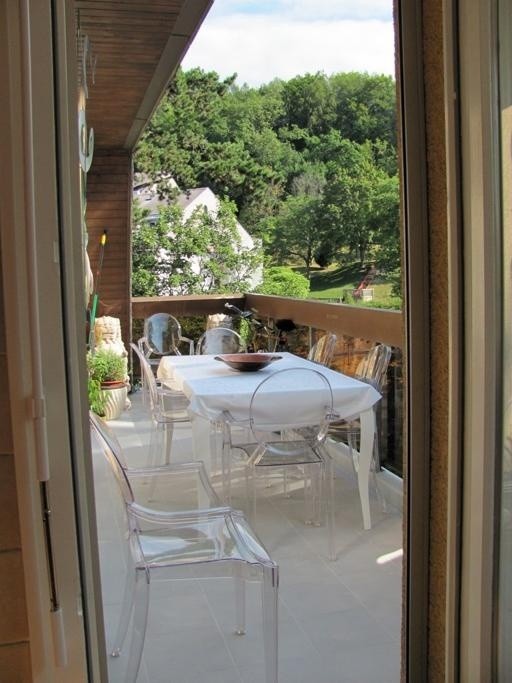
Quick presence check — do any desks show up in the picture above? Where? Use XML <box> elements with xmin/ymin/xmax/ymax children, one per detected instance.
<box><xmin>156</xmin><ymin>352</ymin><xmax>383</xmax><ymax>530</ymax></box>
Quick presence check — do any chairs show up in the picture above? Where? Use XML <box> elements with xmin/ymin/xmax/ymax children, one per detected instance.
<box><xmin>89</xmin><ymin>411</ymin><xmax>279</xmax><ymax>682</ymax></box>
<box><xmin>223</xmin><ymin>335</ymin><xmax>392</xmax><ymax>561</ymax></box>
<box><xmin>130</xmin><ymin>312</ymin><xmax>244</xmax><ymax>466</ymax></box>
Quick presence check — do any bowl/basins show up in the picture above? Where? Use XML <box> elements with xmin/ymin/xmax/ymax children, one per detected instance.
<box><xmin>214</xmin><ymin>353</ymin><xmax>283</xmax><ymax>372</ymax></box>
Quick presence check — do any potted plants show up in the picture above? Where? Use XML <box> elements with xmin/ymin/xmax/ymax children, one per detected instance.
<box><xmin>86</xmin><ymin>331</ymin><xmax>129</xmax><ymax>420</ymax></box>
